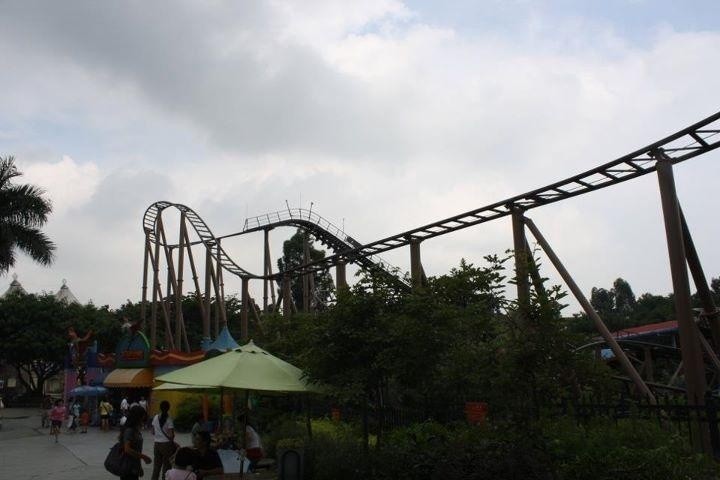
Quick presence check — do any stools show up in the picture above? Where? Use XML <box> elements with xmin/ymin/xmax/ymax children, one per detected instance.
<box><xmin>254</xmin><ymin>457</ymin><xmax>276</xmax><ymax>469</ymax></box>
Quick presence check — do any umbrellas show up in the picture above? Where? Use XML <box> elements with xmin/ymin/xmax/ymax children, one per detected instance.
<box><xmin>154</xmin><ymin>338</ymin><xmax>326</xmax><ymax>479</ymax></box>
<box><xmin>152</xmin><ymin>383</ymin><xmax>225</xmax><ymax>435</ymax></box>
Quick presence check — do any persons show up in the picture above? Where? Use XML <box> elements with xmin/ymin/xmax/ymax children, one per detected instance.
<box><xmin>41</xmin><ymin>394</ymin><xmax>263</xmax><ymax>480</ymax></box>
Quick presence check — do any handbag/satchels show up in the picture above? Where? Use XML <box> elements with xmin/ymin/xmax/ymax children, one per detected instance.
<box><xmin>104</xmin><ymin>443</ymin><xmax>132</xmax><ymax>475</ymax></box>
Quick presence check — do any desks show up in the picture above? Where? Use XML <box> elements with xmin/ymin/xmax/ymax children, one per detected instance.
<box><xmin>217</xmin><ymin>447</ymin><xmax>251</xmax><ymax>474</ymax></box>
